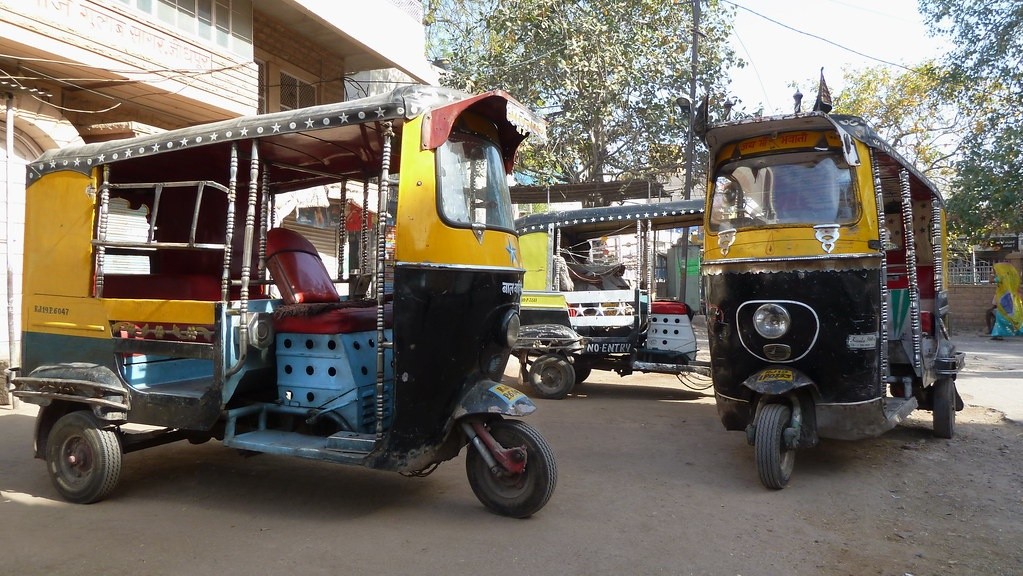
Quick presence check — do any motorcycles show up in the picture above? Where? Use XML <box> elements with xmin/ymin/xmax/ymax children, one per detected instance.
<box><xmin>698</xmin><ymin>110</ymin><xmax>965</xmax><ymax>490</ymax></box>
<box><xmin>511</xmin><ymin>199</ymin><xmax>715</xmax><ymax>400</ymax></box>
<box><xmin>7</xmin><ymin>81</ymin><xmax>556</xmax><ymax>519</ymax></box>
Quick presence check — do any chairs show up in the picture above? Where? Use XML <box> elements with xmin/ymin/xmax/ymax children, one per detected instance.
<box><xmin>265</xmin><ymin>227</ymin><xmax>394</xmax><ymax>335</ymax></box>
<box><xmin>642</xmin><ymin>262</ymin><xmax>688</xmax><ymax>314</ymax></box>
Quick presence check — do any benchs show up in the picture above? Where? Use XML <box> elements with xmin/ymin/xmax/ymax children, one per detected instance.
<box><xmin>103</xmin><ymin>272</ymin><xmax>270</xmax><ymax>366</ymax></box>
<box><xmin>916</xmin><ymin>265</ymin><xmax>935</xmax><ymax>336</ymax></box>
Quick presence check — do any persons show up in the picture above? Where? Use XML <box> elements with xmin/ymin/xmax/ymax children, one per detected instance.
<box><xmin>553</xmin><ymin>238</ymin><xmax>630</xmax><ymax>291</ymax></box>
<box><xmin>985</xmin><ymin>268</ymin><xmax>999</xmax><ymax>334</ymax></box>
